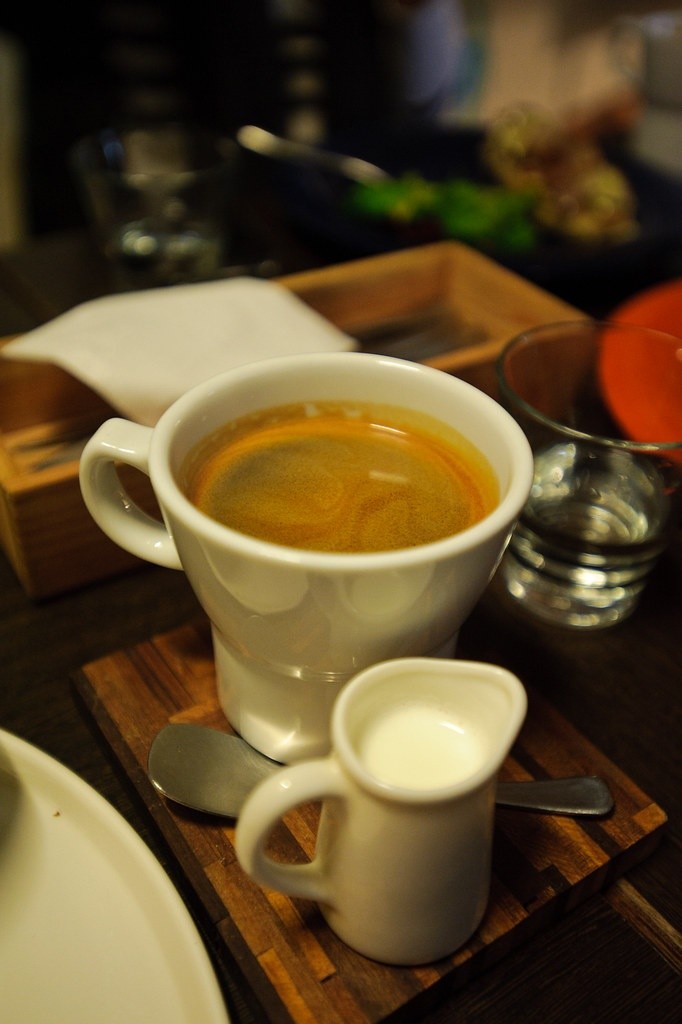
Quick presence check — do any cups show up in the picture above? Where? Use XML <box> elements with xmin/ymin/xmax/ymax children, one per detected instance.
<box><xmin>77</xmin><ymin>350</ymin><xmax>534</xmax><ymax>765</ymax></box>
<box><xmin>234</xmin><ymin>658</ymin><xmax>528</xmax><ymax>966</ymax></box>
<box><xmin>495</xmin><ymin>320</ymin><xmax>682</xmax><ymax>633</ymax></box>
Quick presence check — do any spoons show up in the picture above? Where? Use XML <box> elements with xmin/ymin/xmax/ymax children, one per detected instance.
<box><xmin>147</xmin><ymin>722</ymin><xmax>613</xmax><ymax>817</ymax></box>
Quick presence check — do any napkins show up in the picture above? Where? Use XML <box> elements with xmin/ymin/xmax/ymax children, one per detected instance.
<box><xmin>0</xmin><ymin>277</ymin><xmax>356</xmax><ymax>427</ymax></box>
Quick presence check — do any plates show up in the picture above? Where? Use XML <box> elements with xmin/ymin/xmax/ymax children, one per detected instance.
<box><xmin>0</xmin><ymin>727</ymin><xmax>231</xmax><ymax>1024</ymax></box>
<box><xmin>599</xmin><ymin>284</ymin><xmax>682</xmax><ymax>466</ymax></box>
